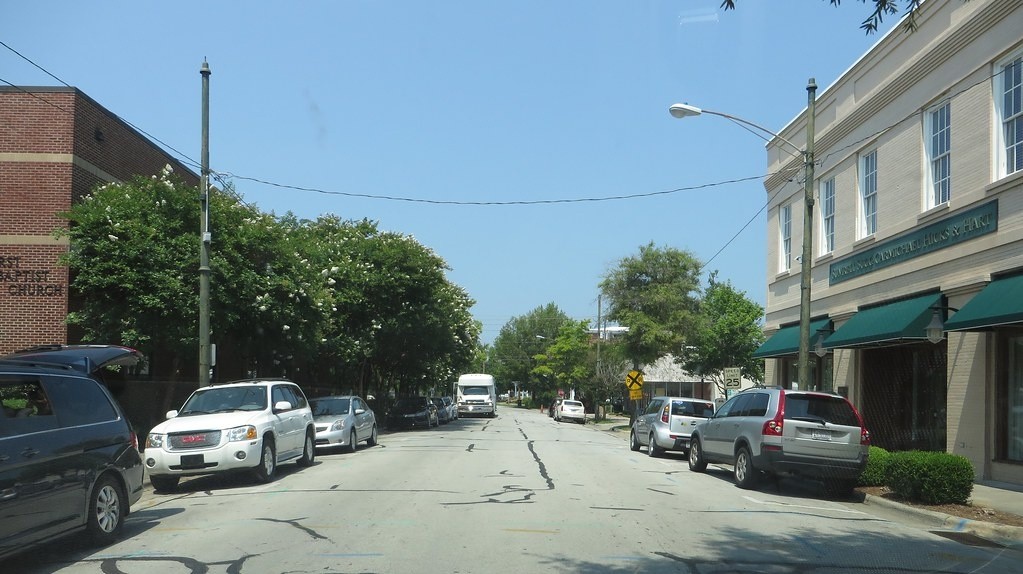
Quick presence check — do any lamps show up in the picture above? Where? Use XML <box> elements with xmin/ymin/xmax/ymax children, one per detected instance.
<box><xmin>922</xmin><ymin>301</ymin><xmax>959</xmax><ymax>345</ymax></box>
<box><xmin>813</xmin><ymin>329</ymin><xmax>836</xmax><ymax>358</ymax></box>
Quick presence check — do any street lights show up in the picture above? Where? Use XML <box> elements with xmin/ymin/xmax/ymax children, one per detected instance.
<box><xmin>669</xmin><ymin>77</ymin><xmax>818</xmax><ymax>392</ymax></box>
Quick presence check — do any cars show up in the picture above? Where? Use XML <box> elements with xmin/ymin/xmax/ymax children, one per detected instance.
<box><xmin>554</xmin><ymin>400</ymin><xmax>586</xmax><ymax>424</ymax></box>
<box><xmin>308</xmin><ymin>396</ymin><xmax>377</xmax><ymax>453</ymax></box>
<box><xmin>430</xmin><ymin>397</ymin><xmax>448</xmax><ymax>425</ymax></box>
<box><xmin>441</xmin><ymin>397</ymin><xmax>458</xmax><ymax>421</ymax></box>
<box><xmin>548</xmin><ymin>398</ymin><xmax>564</xmax><ymax>417</ymax></box>
<box><xmin>386</xmin><ymin>396</ymin><xmax>440</xmax><ymax>431</ymax></box>
<box><xmin>629</xmin><ymin>396</ymin><xmax>716</xmax><ymax>458</ymax></box>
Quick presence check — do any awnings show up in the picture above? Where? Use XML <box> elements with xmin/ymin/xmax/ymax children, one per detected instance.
<box><xmin>942</xmin><ymin>274</ymin><xmax>1023</xmax><ymax>336</ymax></box>
<box><xmin>750</xmin><ymin>318</ymin><xmax>836</xmax><ymax>359</ymax></box>
<box><xmin>823</xmin><ymin>291</ymin><xmax>946</xmax><ymax>350</ymax></box>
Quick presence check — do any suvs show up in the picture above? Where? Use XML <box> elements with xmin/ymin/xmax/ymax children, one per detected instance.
<box><xmin>687</xmin><ymin>384</ymin><xmax>871</xmax><ymax>499</ymax></box>
<box><xmin>0</xmin><ymin>340</ymin><xmax>146</xmax><ymax>566</ymax></box>
<box><xmin>145</xmin><ymin>377</ymin><xmax>318</xmax><ymax>493</ymax></box>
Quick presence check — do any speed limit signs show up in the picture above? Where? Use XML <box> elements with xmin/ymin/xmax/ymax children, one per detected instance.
<box><xmin>723</xmin><ymin>367</ymin><xmax>741</xmax><ymax>390</ymax></box>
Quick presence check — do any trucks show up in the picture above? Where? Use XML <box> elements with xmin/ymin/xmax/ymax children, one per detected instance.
<box><xmin>457</xmin><ymin>373</ymin><xmax>497</xmax><ymax>417</ymax></box>
